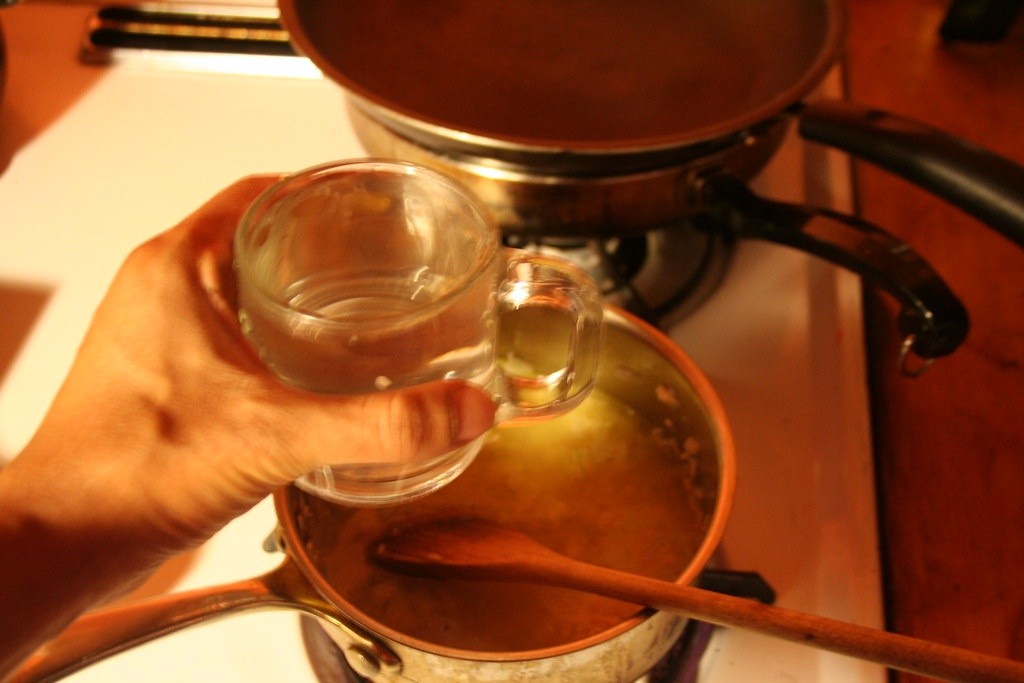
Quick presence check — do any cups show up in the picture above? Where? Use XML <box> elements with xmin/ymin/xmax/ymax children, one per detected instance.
<box><xmin>236</xmin><ymin>162</ymin><xmax>598</xmax><ymax>511</ymax></box>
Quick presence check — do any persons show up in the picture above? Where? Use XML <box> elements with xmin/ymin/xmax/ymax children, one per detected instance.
<box><xmin>0</xmin><ymin>173</ymin><xmax>497</xmax><ymax>682</ymax></box>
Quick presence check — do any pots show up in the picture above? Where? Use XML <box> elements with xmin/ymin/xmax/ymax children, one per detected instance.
<box><xmin>335</xmin><ymin>97</ymin><xmax>969</xmax><ymax>380</ymax></box>
<box><xmin>0</xmin><ymin>308</ymin><xmax>738</xmax><ymax>682</ymax></box>
<box><xmin>276</xmin><ymin>1</ymin><xmax>1023</xmax><ymax>250</ymax></box>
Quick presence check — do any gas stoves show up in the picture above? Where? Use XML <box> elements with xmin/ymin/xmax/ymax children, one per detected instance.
<box><xmin>0</xmin><ymin>44</ymin><xmax>891</xmax><ymax>682</ymax></box>
